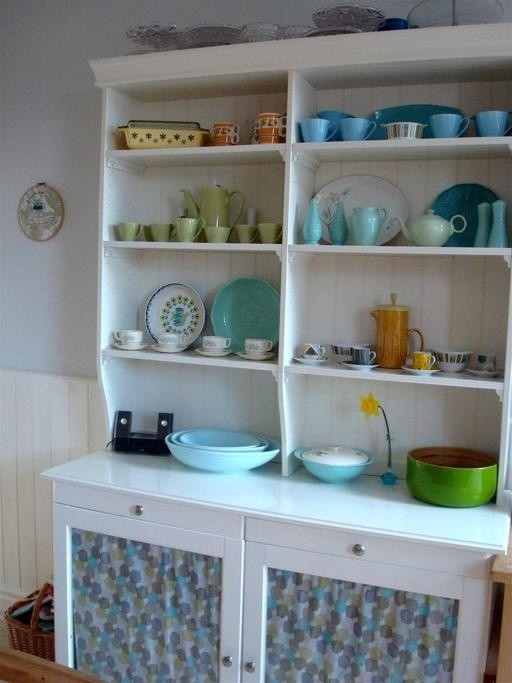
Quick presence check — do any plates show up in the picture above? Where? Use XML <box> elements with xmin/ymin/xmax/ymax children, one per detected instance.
<box><xmin>14</xmin><ymin>181</ymin><xmax>66</xmax><ymax>243</ymax></box>
<box><xmin>143</xmin><ymin>282</ymin><xmax>207</xmax><ymax>349</ymax></box>
<box><xmin>425</xmin><ymin>180</ymin><xmax>502</xmax><ymax>246</ymax></box>
<box><xmin>209</xmin><ymin>274</ymin><xmax>279</xmax><ymax>354</ymax></box>
<box><xmin>111</xmin><ymin>342</ymin><xmax>274</xmax><ymax>360</ymax></box>
<box><xmin>294</xmin><ymin>356</ymin><xmax>502</xmax><ymax>376</ymax></box>
<box><xmin>115</xmin><ymin>0</ymin><xmax>506</xmax><ymax>54</ymax></box>
<box><xmin>305</xmin><ymin>171</ymin><xmax>411</xmax><ymax>244</ymax></box>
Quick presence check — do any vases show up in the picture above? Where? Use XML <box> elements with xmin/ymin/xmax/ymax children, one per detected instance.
<box><xmin>380</xmin><ymin>467</ymin><xmax>397</xmax><ymax>484</ymax></box>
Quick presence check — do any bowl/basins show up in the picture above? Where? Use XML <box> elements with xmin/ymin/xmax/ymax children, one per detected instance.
<box><xmin>163</xmin><ymin>425</ymin><xmax>282</xmax><ymax>476</ymax></box>
<box><xmin>404</xmin><ymin>444</ymin><xmax>497</xmax><ymax>509</ymax></box>
<box><xmin>293</xmin><ymin>437</ymin><xmax>376</xmax><ymax>484</ymax></box>
<box><xmin>116</xmin><ymin>114</ymin><xmax>212</xmax><ymax>148</ymax></box>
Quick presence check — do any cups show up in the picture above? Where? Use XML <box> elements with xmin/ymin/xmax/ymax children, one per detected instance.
<box><xmin>114</xmin><ymin>328</ymin><xmax>273</xmax><ymax>353</ymax></box>
<box><xmin>117</xmin><ymin>217</ymin><xmax>282</xmax><ymax>243</ymax></box>
<box><xmin>299</xmin><ymin>104</ymin><xmax>512</xmax><ymax>144</ymax></box>
<box><xmin>302</xmin><ymin>342</ymin><xmax>497</xmax><ymax>375</ymax></box>
<box><xmin>210</xmin><ymin>112</ymin><xmax>285</xmax><ymax>144</ymax></box>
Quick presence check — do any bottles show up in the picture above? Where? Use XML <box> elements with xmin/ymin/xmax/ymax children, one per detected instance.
<box><xmin>472</xmin><ymin>201</ymin><xmax>510</xmax><ymax>246</ymax></box>
<box><xmin>303</xmin><ymin>199</ymin><xmax>348</xmax><ymax>244</ymax></box>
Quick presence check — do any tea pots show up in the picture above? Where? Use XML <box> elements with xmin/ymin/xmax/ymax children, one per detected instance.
<box><xmin>349</xmin><ymin>206</ymin><xmax>466</xmax><ymax>246</ymax></box>
<box><xmin>369</xmin><ymin>290</ymin><xmax>425</xmax><ymax>373</ymax></box>
<box><xmin>181</xmin><ymin>183</ymin><xmax>244</xmax><ymax>242</ymax></box>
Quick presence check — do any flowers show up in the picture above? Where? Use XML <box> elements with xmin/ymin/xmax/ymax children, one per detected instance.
<box><xmin>361</xmin><ymin>394</ymin><xmax>392</xmax><ymax>467</ymax></box>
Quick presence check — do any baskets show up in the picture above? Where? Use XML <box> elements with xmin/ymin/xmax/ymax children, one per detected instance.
<box><xmin>4</xmin><ymin>582</ymin><xmax>54</xmax><ymax>660</ymax></box>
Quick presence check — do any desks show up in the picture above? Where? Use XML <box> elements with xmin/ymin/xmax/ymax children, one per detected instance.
<box><xmin>490</xmin><ymin>528</ymin><xmax>512</xmax><ymax>683</ymax></box>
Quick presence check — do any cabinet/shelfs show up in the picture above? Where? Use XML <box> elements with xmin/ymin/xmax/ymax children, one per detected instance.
<box><xmin>52</xmin><ymin>479</ymin><xmax>512</xmax><ymax>683</ymax></box>
<box><xmin>86</xmin><ymin>22</ymin><xmax>511</xmax><ymax>509</ymax></box>
